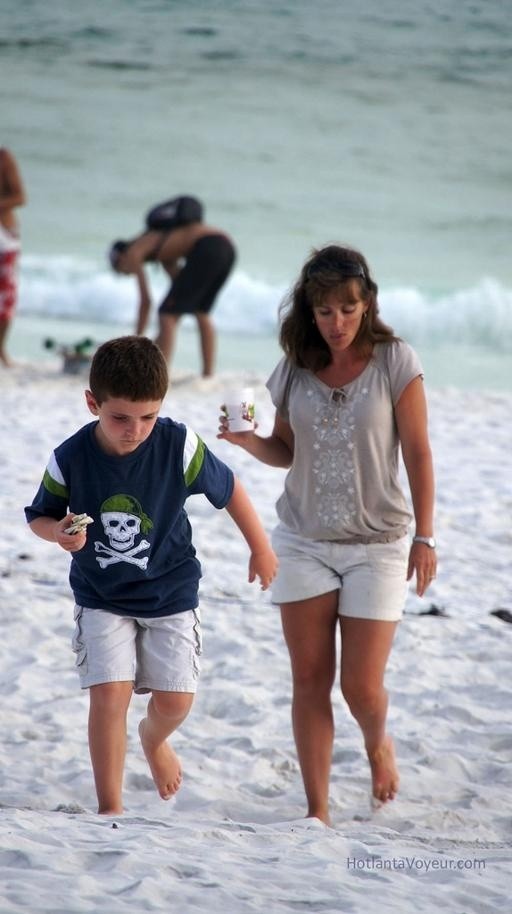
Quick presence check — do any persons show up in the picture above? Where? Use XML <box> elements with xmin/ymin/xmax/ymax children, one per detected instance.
<box><xmin>1</xmin><ymin>146</ymin><xmax>26</xmax><ymax>368</ymax></box>
<box><xmin>109</xmin><ymin>193</ymin><xmax>237</xmax><ymax>381</ymax></box>
<box><xmin>23</xmin><ymin>333</ymin><xmax>282</xmax><ymax>816</ymax></box>
<box><xmin>215</xmin><ymin>242</ymin><xmax>441</xmax><ymax>828</ymax></box>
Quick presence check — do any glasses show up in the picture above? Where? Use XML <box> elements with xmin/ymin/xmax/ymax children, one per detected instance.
<box><xmin>310</xmin><ymin>257</ymin><xmax>369</xmax><ymax>282</ymax></box>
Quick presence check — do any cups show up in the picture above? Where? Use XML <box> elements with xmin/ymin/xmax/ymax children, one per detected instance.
<box><xmin>223</xmin><ymin>385</ymin><xmax>257</xmax><ymax>434</ymax></box>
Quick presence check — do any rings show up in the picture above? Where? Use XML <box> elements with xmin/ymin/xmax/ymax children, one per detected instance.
<box><xmin>427</xmin><ymin>575</ymin><xmax>436</xmax><ymax>579</ymax></box>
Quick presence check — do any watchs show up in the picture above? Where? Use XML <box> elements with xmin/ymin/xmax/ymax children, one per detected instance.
<box><xmin>411</xmin><ymin>535</ymin><xmax>438</xmax><ymax>549</ymax></box>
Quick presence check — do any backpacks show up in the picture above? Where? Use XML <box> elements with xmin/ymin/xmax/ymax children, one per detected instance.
<box><xmin>141</xmin><ymin>193</ymin><xmax>206</xmax><ymax>262</ymax></box>
